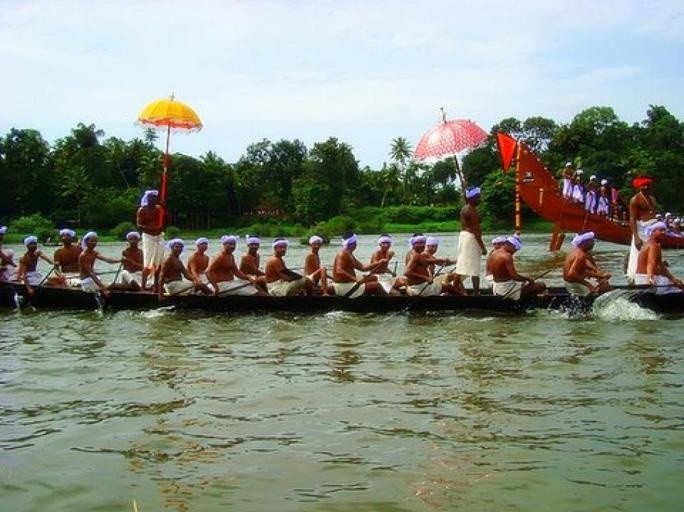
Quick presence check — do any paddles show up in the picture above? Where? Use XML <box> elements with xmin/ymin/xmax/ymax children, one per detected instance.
<box><xmin>97</xmin><ymin>261</ymin><xmax>122</xmax><ymax>314</ymax></box>
<box><xmin>616</xmin><ymin>283</ymin><xmax>676</xmax><ymax>290</ymax></box>
<box><xmin>562</xmin><ymin>277</ymin><xmax>606</xmax><ymax>313</ymax></box>
<box><xmin>345</xmin><ymin>263</ymin><xmax>380</xmax><ymax>296</ymax></box>
<box><xmin>19</xmin><ymin>266</ymin><xmax>56</xmax><ymax>311</ymax></box>
<box><xmin>402</xmin><ymin>262</ymin><xmax>446</xmax><ymax>312</ymax></box>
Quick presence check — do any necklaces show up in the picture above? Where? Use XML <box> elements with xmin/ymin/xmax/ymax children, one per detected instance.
<box><xmin>639</xmin><ymin>192</ymin><xmax>654</xmax><ymax>215</ymax></box>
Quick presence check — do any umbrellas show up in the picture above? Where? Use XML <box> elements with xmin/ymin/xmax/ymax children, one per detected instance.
<box><xmin>413</xmin><ymin>106</ymin><xmax>489</xmax><ymax>205</ymax></box>
<box><xmin>134</xmin><ymin>91</ymin><xmax>201</xmax><ymax>226</ymax></box>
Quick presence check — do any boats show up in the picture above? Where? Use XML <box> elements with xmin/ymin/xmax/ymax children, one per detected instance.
<box><xmin>0</xmin><ymin>274</ymin><xmax>684</xmax><ymax>312</ymax></box>
<box><xmin>517</xmin><ymin>141</ymin><xmax>684</xmax><ymax>252</ymax></box>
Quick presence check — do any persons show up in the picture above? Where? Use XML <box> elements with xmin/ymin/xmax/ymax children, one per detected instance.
<box><xmin>369</xmin><ymin>233</ymin><xmax>405</xmax><ymax>295</ymax></box>
<box><xmin>484</xmin><ymin>236</ymin><xmax>507</xmax><ymax>289</ymax></box>
<box><xmin>238</xmin><ymin>236</ymin><xmax>265</xmax><ymax>276</ymax></box>
<box><xmin>626</xmin><ymin>177</ymin><xmax>657</xmax><ymax>285</ymax></box>
<box><xmin>562</xmin><ymin>232</ymin><xmax>611</xmax><ymax>297</ymax></box>
<box><xmin>205</xmin><ymin>235</ymin><xmax>266</xmax><ymax>297</ymax></box>
<box><xmin>655</xmin><ymin>211</ymin><xmax>684</xmax><ymax>238</ymax></box>
<box><xmin>121</xmin><ymin>231</ymin><xmax>155</xmax><ymax>293</ymax></box>
<box><xmin>420</xmin><ymin>236</ymin><xmax>467</xmax><ymax>296</ymax></box>
<box><xmin>0</xmin><ymin>226</ymin><xmax>16</xmax><ymax>282</ymax></box>
<box><xmin>635</xmin><ymin>223</ymin><xmax>683</xmax><ymax>288</ymax></box>
<box><xmin>490</xmin><ymin>235</ymin><xmax>545</xmax><ymax>296</ymax></box>
<box><xmin>54</xmin><ymin>228</ymin><xmax>82</xmax><ymax>288</ymax></box>
<box><xmin>264</xmin><ymin>238</ymin><xmax>313</xmax><ymax>297</ymax></box>
<box><xmin>332</xmin><ymin>231</ymin><xmax>387</xmax><ymax>298</ymax></box>
<box><xmin>187</xmin><ymin>236</ymin><xmax>209</xmax><ymax>282</ymax></box>
<box><xmin>303</xmin><ymin>236</ymin><xmax>327</xmax><ymax>296</ymax></box>
<box><xmin>77</xmin><ymin>231</ymin><xmax>124</xmax><ymax>295</ymax></box>
<box><xmin>11</xmin><ymin>234</ymin><xmax>58</xmax><ymax>294</ymax></box>
<box><xmin>560</xmin><ymin>162</ymin><xmax>629</xmax><ymax>228</ymax></box>
<box><xmin>403</xmin><ymin>232</ymin><xmax>463</xmax><ymax>296</ymax></box>
<box><xmin>455</xmin><ymin>187</ymin><xmax>487</xmax><ymax>295</ymax></box>
<box><xmin>135</xmin><ymin>190</ymin><xmax>166</xmax><ymax>292</ymax></box>
<box><xmin>157</xmin><ymin>238</ymin><xmax>211</xmax><ymax>301</ymax></box>
